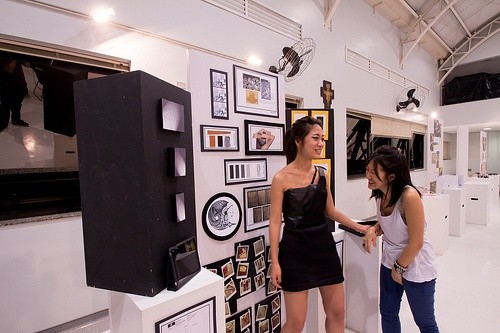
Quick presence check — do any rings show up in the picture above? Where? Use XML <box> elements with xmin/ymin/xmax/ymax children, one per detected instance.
<box><xmin>363</xmin><ymin>243</ymin><xmax>365</xmax><ymax>246</ymax></box>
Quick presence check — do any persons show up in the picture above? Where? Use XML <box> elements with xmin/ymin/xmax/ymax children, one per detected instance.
<box><xmin>0</xmin><ymin>50</ymin><xmax>29</xmax><ymax>132</ymax></box>
<box><xmin>362</xmin><ymin>146</ymin><xmax>440</xmax><ymax>333</ymax></box>
<box><xmin>269</xmin><ymin>116</ymin><xmax>384</xmax><ymax>333</ymax></box>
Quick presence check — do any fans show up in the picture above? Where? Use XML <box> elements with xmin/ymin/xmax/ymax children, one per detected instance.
<box><xmin>396</xmin><ymin>85</ymin><xmax>425</xmax><ymax>112</ymax></box>
<box><xmin>269</xmin><ymin>38</ymin><xmax>316</xmax><ymax>82</ymax></box>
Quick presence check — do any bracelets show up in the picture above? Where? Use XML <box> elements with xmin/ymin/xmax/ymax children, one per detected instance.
<box><xmin>394</xmin><ymin>260</ymin><xmax>407</xmax><ymax>271</ymax></box>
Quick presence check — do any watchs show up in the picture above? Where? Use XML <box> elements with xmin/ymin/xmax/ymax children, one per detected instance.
<box><xmin>394</xmin><ymin>264</ymin><xmax>404</xmax><ymax>274</ymax></box>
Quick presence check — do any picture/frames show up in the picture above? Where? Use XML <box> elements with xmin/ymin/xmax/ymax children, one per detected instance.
<box><xmin>200</xmin><ymin>64</ymin><xmax>287</xmax><ymax>240</ymax></box>
